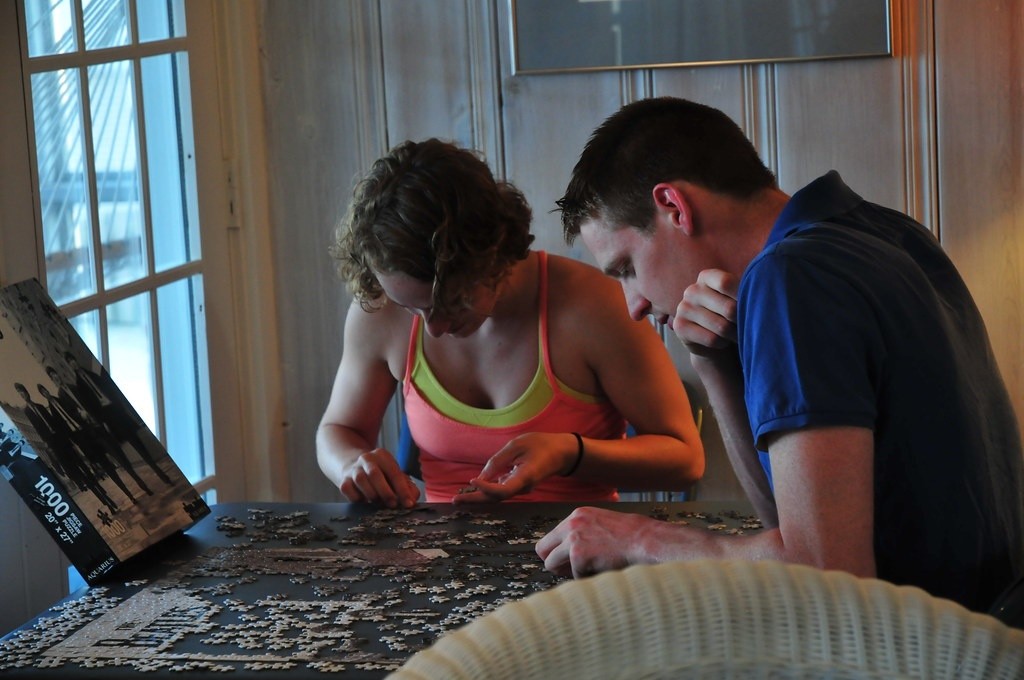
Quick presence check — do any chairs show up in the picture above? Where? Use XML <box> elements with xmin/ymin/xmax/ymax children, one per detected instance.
<box><xmin>392</xmin><ymin>377</ymin><xmax>703</xmax><ymax>501</ymax></box>
<box><xmin>379</xmin><ymin>558</ymin><xmax>1024</xmax><ymax>680</ymax></box>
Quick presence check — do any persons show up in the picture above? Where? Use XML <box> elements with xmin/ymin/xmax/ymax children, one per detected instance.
<box><xmin>534</xmin><ymin>97</ymin><xmax>1024</xmax><ymax>628</ymax></box>
<box><xmin>315</xmin><ymin>138</ymin><xmax>705</xmax><ymax>506</ymax></box>
<box><xmin>15</xmin><ymin>351</ymin><xmax>172</xmax><ymax>515</ymax></box>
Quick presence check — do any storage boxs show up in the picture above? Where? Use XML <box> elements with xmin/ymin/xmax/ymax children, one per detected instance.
<box><xmin>0</xmin><ymin>277</ymin><xmax>211</xmax><ymax>587</ymax></box>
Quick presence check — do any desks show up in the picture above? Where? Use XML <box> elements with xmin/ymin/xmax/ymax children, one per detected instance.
<box><xmin>0</xmin><ymin>499</ymin><xmax>762</xmax><ymax>680</ymax></box>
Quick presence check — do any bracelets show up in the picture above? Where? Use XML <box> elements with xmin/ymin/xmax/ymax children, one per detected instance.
<box><xmin>561</xmin><ymin>432</ymin><xmax>584</xmax><ymax>477</ymax></box>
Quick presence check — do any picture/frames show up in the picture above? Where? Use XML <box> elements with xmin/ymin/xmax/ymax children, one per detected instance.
<box><xmin>507</xmin><ymin>0</ymin><xmax>893</xmax><ymax>76</ymax></box>
